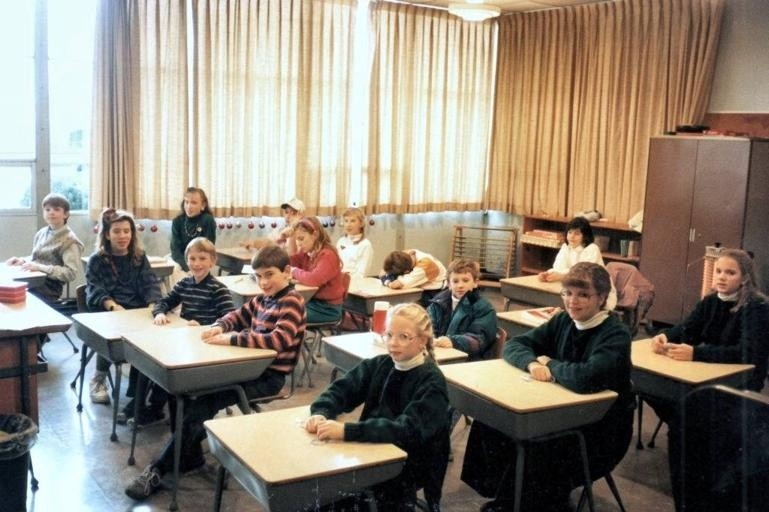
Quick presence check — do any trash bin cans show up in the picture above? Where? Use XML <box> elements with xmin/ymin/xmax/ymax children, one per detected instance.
<box><xmin>0</xmin><ymin>413</ymin><xmax>38</xmax><ymax>512</ymax></box>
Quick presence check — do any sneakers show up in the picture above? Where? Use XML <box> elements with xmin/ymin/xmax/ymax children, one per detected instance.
<box><xmin>126</xmin><ymin>464</ymin><xmax>162</xmax><ymax>500</ymax></box>
<box><xmin>90</xmin><ymin>374</ymin><xmax>110</xmax><ymax>403</ymax></box>
<box><xmin>118</xmin><ymin>401</ymin><xmax>166</xmax><ymax>430</ymax></box>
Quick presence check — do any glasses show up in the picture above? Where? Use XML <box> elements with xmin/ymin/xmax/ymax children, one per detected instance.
<box><xmin>559</xmin><ymin>288</ymin><xmax>599</xmax><ymax>303</ymax></box>
<box><xmin>381</xmin><ymin>328</ymin><xmax>422</xmax><ymax>345</ymax></box>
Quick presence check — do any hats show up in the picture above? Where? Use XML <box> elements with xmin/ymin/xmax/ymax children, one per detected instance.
<box><xmin>281</xmin><ymin>199</ymin><xmax>304</xmax><ymax>211</ymax></box>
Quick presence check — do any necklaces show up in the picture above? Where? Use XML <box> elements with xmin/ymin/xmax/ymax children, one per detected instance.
<box><xmin>110</xmin><ymin>257</ymin><xmax>133</xmax><ymax>285</ymax></box>
<box><xmin>185</xmin><ymin>216</ymin><xmax>198</xmax><ymax>238</ymax></box>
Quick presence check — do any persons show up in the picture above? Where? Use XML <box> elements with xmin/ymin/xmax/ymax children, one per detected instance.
<box><xmin>6</xmin><ymin>193</ymin><xmax>85</xmax><ymax>354</ymax></box>
<box><xmin>479</xmin><ymin>262</ymin><xmax>635</xmax><ymax>512</ymax></box>
<box><xmin>86</xmin><ymin>208</ymin><xmax>163</xmax><ymax>403</ymax></box>
<box><xmin>124</xmin><ymin>245</ymin><xmax>306</xmax><ymax>499</ymax></box>
<box><xmin>305</xmin><ymin>301</ymin><xmax>450</xmax><ymax>512</ymax></box>
<box><xmin>284</xmin><ymin>216</ymin><xmax>345</xmax><ymax>323</ymax></box>
<box><xmin>537</xmin><ymin>216</ymin><xmax>618</xmax><ymax>312</ymax></box>
<box><xmin>335</xmin><ymin>208</ymin><xmax>371</xmax><ymax>279</ymax></box>
<box><xmin>241</xmin><ymin>198</ymin><xmax>305</xmax><ymax>255</ymax></box>
<box><xmin>380</xmin><ymin>249</ymin><xmax>449</xmax><ymax>310</ymax></box>
<box><xmin>170</xmin><ymin>188</ymin><xmax>217</xmax><ymax>311</ymax></box>
<box><xmin>650</xmin><ymin>248</ymin><xmax>769</xmax><ymax>512</ymax></box>
<box><xmin>116</xmin><ymin>236</ymin><xmax>235</xmax><ymax>431</ymax></box>
<box><xmin>423</xmin><ymin>256</ymin><xmax>497</xmax><ymax>462</ymax></box>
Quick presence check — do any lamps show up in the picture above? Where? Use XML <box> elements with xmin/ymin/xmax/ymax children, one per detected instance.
<box><xmin>446</xmin><ymin>0</ymin><xmax>503</xmax><ymax>21</ymax></box>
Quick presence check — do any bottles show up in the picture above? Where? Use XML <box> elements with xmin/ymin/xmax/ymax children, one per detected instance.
<box><xmin>373</xmin><ymin>301</ymin><xmax>387</xmax><ymax>333</ymax></box>
<box><xmin>619</xmin><ymin>238</ymin><xmax>641</xmax><ymax>257</ymax></box>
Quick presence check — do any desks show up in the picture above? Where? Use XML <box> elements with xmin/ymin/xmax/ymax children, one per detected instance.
<box><xmin>3</xmin><ymin>263</ymin><xmax>48</xmax><ymax>288</ymax></box>
<box><xmin>493</xmin><ymin>274</ymin><xmax>565</xmax><ymax>312</ymax></box>
<box><xmin>71</xmin><ymin>307</ymin><xmax>190</xmax><ymax>441</ymax></box>
<box><xmin>81</xmin><ymin>255</ymin><xmax>175</xmax><ymax>288</ymax></box>
<box><xmin>437</xmin><ymin>359</ymin><xmax>620</xmax><ymax>512</ymax></box>
<box><xmin>2</xmin><ymin>291</ymin><xmax>74</xmax><ymax>433</ymax></box>
<box><xmin>216</xmin><ymin>275</ymin><xmax>319</xmax><ymax>394</ymax></box>
<box><xmin>496</xmin><ymin>307</ymin><xmax>564</xmax><ymax>338</ymax></box>
<box><xmin>322</xmin><ymin>332</ymin><xmax>469</xmax><ymax>384</ymax></box>
<box><xmin>343</xmin><ymin>277</ymin><xmax>422</xmax><ymax>330</ymax></box>
<box><xmin>203</xmin><ymin>404</ymin><xmax>408</xmax><ymax>512</ymax></box>
<box><xmin>631</xmin><ymin>338</ymin><xmax>757</xmax><ymax>510</ymax></box>
<box><xmin>117</xmin><ymin>324</ymin><xmax>276</xmax><ymax>511</ymax></box>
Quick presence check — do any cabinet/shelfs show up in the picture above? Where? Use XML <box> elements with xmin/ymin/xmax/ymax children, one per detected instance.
<box><xmin>214</xmin><ymin>244</ymin><xmax>253</xmax><ymax>275</ymax></box>
<box><xmin>516</xmin><ymin>214</ymin><xmax>642</xmax><ymax>283</ymax></box>
<box><xmin>640</xmin><ymin>134</ymin><xmax>769</xmax><ymax>335</ymax></box>
